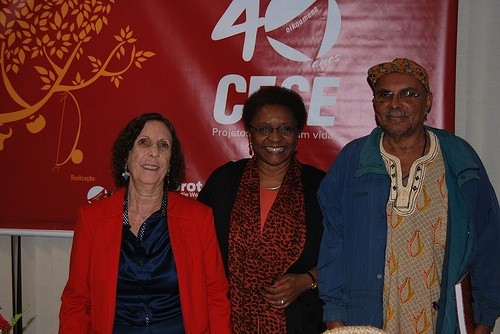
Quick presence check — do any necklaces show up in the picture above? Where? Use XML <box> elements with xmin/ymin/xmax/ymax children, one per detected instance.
<box><xmin>259</xmin><ymin>184</ymin><xmax>281</xmax><ymax>190</ymax></box>
<box><xmin>402</xmin><ymin>128</ymin><xmax>427</xmax><ymax>181</ymax></box>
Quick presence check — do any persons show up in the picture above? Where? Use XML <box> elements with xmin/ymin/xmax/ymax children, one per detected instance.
<box><xmin>316</xmin><ymin>57</ymin><xmax>500</xmax><ymax>334</ymax></box>
<box><xmin>58</xmin><ymin>113</ymin><xmax>231</xmax><ymax>334</ymax></box>
<box><xmin>103</xmin><ymin>86</ymin><xmax>330</xmax><ymax>334</ymax></box>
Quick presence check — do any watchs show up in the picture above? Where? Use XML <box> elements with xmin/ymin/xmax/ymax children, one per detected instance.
<box><xmin>306</xmin><ymin>271</ymin><xmax>317</xmax><ymax>291</ymax></box>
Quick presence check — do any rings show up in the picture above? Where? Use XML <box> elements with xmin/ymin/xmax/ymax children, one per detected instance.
<box><xmin>281</xmin><ymin>299</ymin><xmax>284</xmax><ymax>305</ymax></box>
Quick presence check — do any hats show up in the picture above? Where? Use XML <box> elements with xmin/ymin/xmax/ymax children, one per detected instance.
<box><xmin>366</xmin><ymin>57</ymin><xmax>431</xmax><ymax>94</ymax></box>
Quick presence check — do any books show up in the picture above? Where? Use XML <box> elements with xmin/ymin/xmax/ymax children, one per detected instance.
<box><xmin>455</xmin><ymin>271</ymin><xmax>475</xmax><ymax>334</ymax></box>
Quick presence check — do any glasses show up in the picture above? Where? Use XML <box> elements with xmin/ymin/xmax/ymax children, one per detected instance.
<box><xmin>373</xmin><ymin>87</ymin><xmax>420</xmax><ymax>102</ymax></box>
<box><xmin>250</xmin><ymin>123</ymin><xmax>296</xmax><ymax>137</ymax></box>
